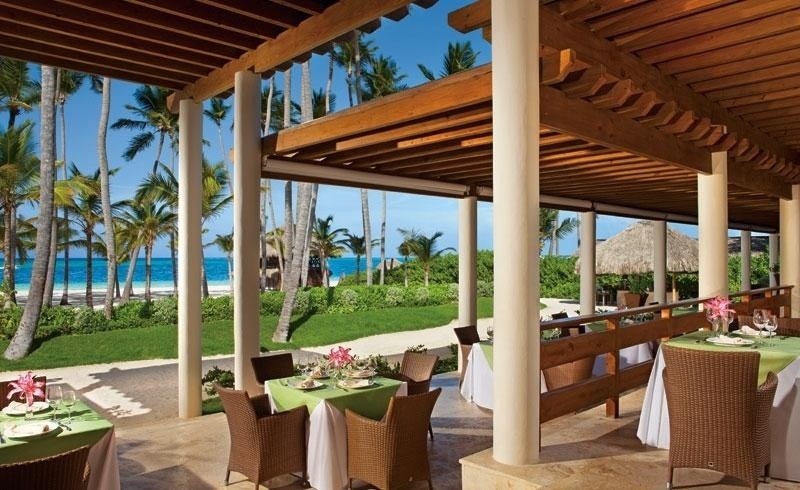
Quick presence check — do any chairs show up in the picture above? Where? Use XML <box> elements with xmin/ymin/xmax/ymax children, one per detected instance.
<box><xmin>344</xmin><ymin>387</ymin><xmax>444</xmax><ymax>490</ymax></box>
<box><xmin>6</xmin><ymin>373</ymin><xmax>49</xmax><ymax>407</ymax></box>
<box><xmin>649</xmin><ymin>301</ymin><xmax>659</xmax><ymax>314</ymax></box>
<box><xmin>250</xmin><ymin>353</ymin><xmax>294</xmax><ymax>393</ymax></box>
<box><xmin>737</xmin><ymin>315</ymin><xmax>800</xmax><ymax>337</ymax></box>
<box><xmin>541</xmin><ymin>328</ymin><xmax>596</xmax><ymax>394</ymax></box>
<box><xmin>0</xmin><ymin>444</ymin><xmax>91</xmax><ymax>490</ymax></box>
<box><xmin>212</xmin><ymin>383</ymin><xmax>309</xmax><ymax>490</ymax></box>
<box><xmin>454</xmin><ymin>325</ymin><xmax>481</xmax><ymax>392</ymax></box>
<box><xmin>551</xmin><ymin>312</ymin><xmax>569</xmax><ymax>320</ymax></box>
<box><xmin>386</xmin><ymin>350</ymin><xmax>439</xmax><ymax>442</ymax></box>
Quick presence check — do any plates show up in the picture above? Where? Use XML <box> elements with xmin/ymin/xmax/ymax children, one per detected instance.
<box><xmin>294</xmin><ymin>383</ymin><xmax>324</xmax><ymax>390</ymax></box>
<box><xmin>706</xmin><ymin>337</ymin><xmax>755</xmax><ymax>347</ymax></box>
<box><xmin>5</xmin><ymin>402</ymin><xmax>50</xmax><ymax>415</ymax></box>
<box><xmin>338</xmin><ymin>379</ymin><xmax>373</xmax><ymax>388</ymax></box>
<box><xmin>732</xmin><ymin>330</ymin><xmax>776</xmax><ymax>337</ymax></box>
<box><xmin>345</xmin><ymin>373</ymin><xmax>375</xmax><ymax>378</ymax></box>
<box><xmin>4</xmin><ymin>421</ymin><xmax>58</xmax><ymax>439</ymax></box>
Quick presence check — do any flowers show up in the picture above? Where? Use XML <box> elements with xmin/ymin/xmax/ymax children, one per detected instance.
<box><xmin>7</xmin><ymin>371</ymin><xmax>44</xmax><ymax>407</ymax></box>
<box><xmin>702</xmin><ymin>296</ymin><xmax>734</xmax><ymax>323</ymax></box>
<box><xmin>327</xmin><ymin>346</ymin><xmax>356</xmax><ymax>372</ymax></box>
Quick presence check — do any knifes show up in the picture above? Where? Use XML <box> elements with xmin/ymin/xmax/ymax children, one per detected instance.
<box><xmin>58</xmin><ymin>422</ymin><xmax>72</xmax><ymax>431</ymax></box>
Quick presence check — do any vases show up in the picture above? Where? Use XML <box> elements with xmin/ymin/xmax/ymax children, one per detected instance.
<box><xmin>711</xmin><ymin>316</ymin><xmax>729</xmax><ymax>335</ymax></box>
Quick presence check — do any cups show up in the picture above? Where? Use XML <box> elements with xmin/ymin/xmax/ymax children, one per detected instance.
<box><xmin>46</xmin><ymin>385</ymin><xmax>62</xmax><ymax>422</ymax></box>
<box><xmin>307</xmin><ymin>356</ymin><xmax>319</xmax><ymax>378</ymax></box>
<box><xmin>753</xmin><ymin>309</ymin><xmax>768</xmax><ymax>344</ymax></box>
<box><xmin>62</xmin><ymin>391</ymin><xmax>76</xmax><ymax>420</ymax></box>
<box><xmin>764</xmin><ymin>315</ymin><xmax>778</xmax><ymax>347</ymax></box>
<box><xmin>298</xmin><ymin>358</ymin><xmax>307</xmax><ymax>376</ymax></box>
